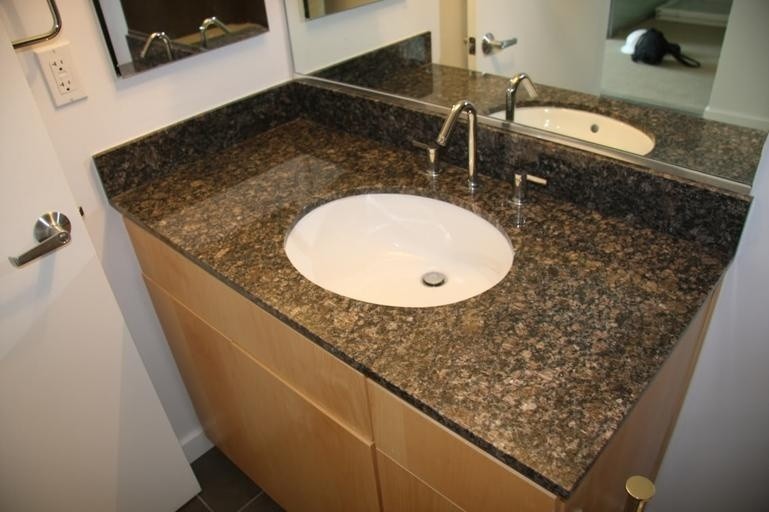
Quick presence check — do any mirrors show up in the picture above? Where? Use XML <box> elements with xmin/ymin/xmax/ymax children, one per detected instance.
<box><xmin>89</xmin><ymin>0</ymin><xmax>270</xmax><ymax>79</ymax></box>
<box><xmin>283</xmin><ymin>0</ymin><xmax>769</xmax><ymax>197</ymax></box>
<box><xmin>304</xmin><ymin>0</ymin><xmax>380</xmax><ymax>19</ymax></box>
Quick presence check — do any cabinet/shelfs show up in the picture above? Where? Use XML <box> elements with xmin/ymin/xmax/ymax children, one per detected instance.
<box><xmin>121</xmin><ymin>215</ymin><xmax>564</xmax><ymax>512</ymax></box>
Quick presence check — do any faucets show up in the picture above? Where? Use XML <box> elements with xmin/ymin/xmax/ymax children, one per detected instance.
<box><xmin>198</xmin><ymin>16</ymin><xmax>232</xmax><ymax>47</ymax></box>
<box><xmin>502</xmin><ymin>73</ymin><xmax>540</xmax><ymax>121</ymax></box>
<box><xmin>140</xmin><ymin>33</ymin><xmax>173</xmax><ymax>63</ymax></box>
<box><xmin>435</xmin><ymin>95</ymin><xmax>482</xmax><ymax>185</ymax></box>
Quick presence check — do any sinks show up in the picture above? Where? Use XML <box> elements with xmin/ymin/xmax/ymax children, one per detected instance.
<box><xmin>488</xmin><ymin>101</ymin><xmax>654</xmax><ymax>158</ymax></box>
<box><xmin>278</xmin><ymin>189</ymin><xmax>516</xmax><ymax>313</ymax></box>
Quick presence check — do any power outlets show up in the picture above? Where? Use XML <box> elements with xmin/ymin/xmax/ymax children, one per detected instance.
<box><xmin>33</xmin><ymin>41</ymin><xmax>87</xmax><ymax>108</ymax></box>
